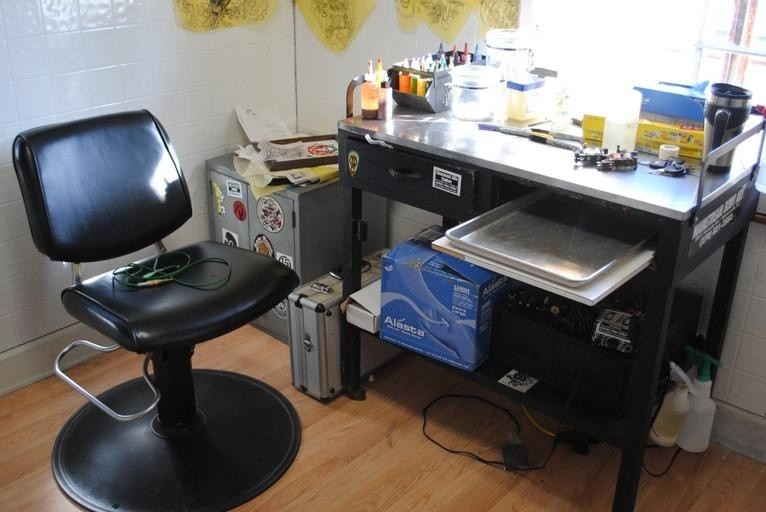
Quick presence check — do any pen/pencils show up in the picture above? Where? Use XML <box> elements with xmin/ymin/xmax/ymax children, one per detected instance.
<box><xmin>477</xmin><ymin>118</ymin><xmax>585</xmax><ymax>152</ymax></box>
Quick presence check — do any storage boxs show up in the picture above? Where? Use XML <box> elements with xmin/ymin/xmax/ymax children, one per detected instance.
<box><xmin>381</xmin><ymin>223</ymin><xmax>512</xmax><ymax>371</ymax></box>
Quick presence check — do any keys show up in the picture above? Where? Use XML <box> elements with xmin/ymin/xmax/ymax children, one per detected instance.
<box><xmin>637</xmin><ymin>156</ymin><xmax>697</xmax><ymax>177</ymax></box>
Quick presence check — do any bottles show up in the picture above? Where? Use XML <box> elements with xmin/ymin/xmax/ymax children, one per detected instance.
<box><xmin>372</xmin><ymin>58</ymin><xmax>388</xmax><ymax>89</ymax></box>
<box><xmin>377</xmin><ymin>81</ymin><xmax>393</xmax><ymax>121</ymax></box>
<box><xmin>360</xmin><ymin>59</ymin><xmax>378</xmax><ymax>119</ymax></box>
<box><xmin>396</xmin><ymin>40</ymin><xmax>482</xmax><ymax>97</ymax></box>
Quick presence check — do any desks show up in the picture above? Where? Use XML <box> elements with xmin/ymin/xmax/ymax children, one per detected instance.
<box><xmin>338</xmin><ymin>113</ymin><xmax>765</xmax><ymax>511</ymax></box>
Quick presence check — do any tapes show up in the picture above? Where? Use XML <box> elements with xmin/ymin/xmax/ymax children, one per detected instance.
<box><xmin>658</xmin><ymin>144</ymin><xmax>681</xmax><ymax>161</ymax></box>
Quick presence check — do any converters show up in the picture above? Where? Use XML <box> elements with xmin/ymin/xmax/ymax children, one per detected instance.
<box><xmin>501</xmin><ymin>443</ymin><xmax>529</xmax><ymax>467</ymax></box>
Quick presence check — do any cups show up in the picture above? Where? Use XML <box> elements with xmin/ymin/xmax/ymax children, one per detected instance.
<box><xmin>701</xmin><ymin>82</ymin><xmax>753</xmax><ymax>175</ymax></box>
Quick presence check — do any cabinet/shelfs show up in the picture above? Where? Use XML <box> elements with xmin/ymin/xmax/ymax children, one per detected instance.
<box><xmin>204</xmin><ymin>148</ymin><xmax>389</xmax><ymax>344</ymax></box>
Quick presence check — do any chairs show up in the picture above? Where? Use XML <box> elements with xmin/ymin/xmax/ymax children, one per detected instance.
<box><xmin>11</xmin><ymin>110</ymin><xmax>301</xmax><ymax>512</ymax></box>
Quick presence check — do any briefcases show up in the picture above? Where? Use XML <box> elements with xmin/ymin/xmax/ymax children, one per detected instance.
<box><xmin>285</xmin><ymin>243</ymin><xmax>419</xmax><ymax>406</ymax></box>
